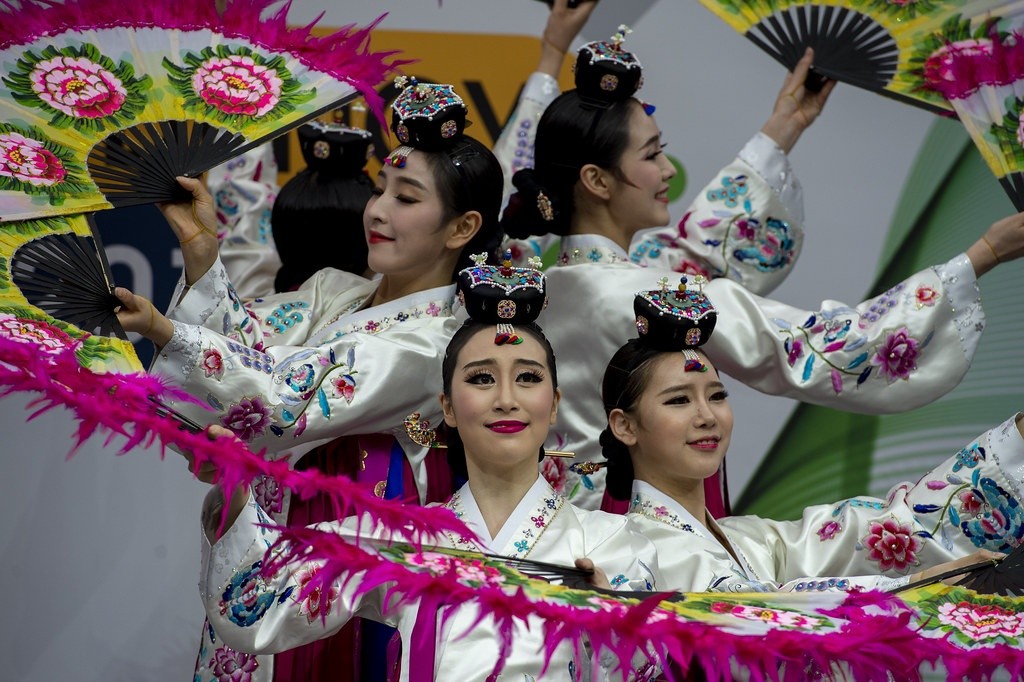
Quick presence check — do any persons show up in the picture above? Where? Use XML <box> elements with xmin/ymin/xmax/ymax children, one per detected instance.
<box><xmin>115</xmin><ymin>76</ymin><xmax>504</xmax><ymax>682</ymax></box>
<box><xmin>206</xmin><ymin>0</ymin><xmax>600</xmax><ymax>298</ymax></box>
<box><xmin>198</xmin><ymin>249</ymin><xmax>668</xmax><ymax>682</ymax></box>
<box><xmin>499</xmin><ymin>24</ymin><xmax>1024</xmax><ymax>515</ymax></box>
<box><xmin>568</xmin><ymin>274</ymin><xmax>1024</xmax><ymax>682</ymax></box>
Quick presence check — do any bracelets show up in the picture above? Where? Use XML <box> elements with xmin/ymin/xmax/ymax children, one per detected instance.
<box><xmin>141</xmin><ymin>303</ymin><xmax>154</xmax><ymax>336</ymax></box>
<box><xmin>544</xmin><ymin>31</ymin><xmax>566</xmax><ymax>55</ymax></box>
<box><xmin>983</xmin><ymin>237</ymin><xmax>1000</xmax><ymax>264</ymax></box>
